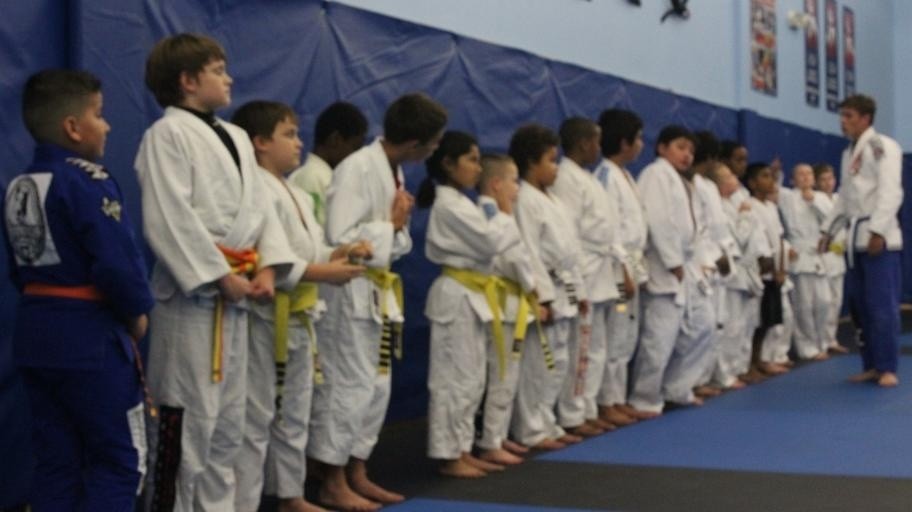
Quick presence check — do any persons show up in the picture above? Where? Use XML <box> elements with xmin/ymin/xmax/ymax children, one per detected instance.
<box><xmin>231</xmin><ymin>99</ymin><xmax>371</xmax><ymax>512</ymax></box>
<box><xmin>292</xmin><ymin>94</ymin><xmax>449</xmax><ymax>511</ymax></box>
<box><xmin>2</xmin><ymin>66</ymin><xmax>154</xmax><ymax>512</ymax></box>
<box><xmin>834</xmin><ymin>97</ymin><xmax>906</xmax><ymax>387</ymax></box>
<box><xmin>415</xmin><ymin>108</ymin><xmax>851</xmax><ymax>478</ymax></box>
<box><xmin>135</xmin><ymin>32</ymin><xmax>275</xmax><ymax>511</ymax></box>
